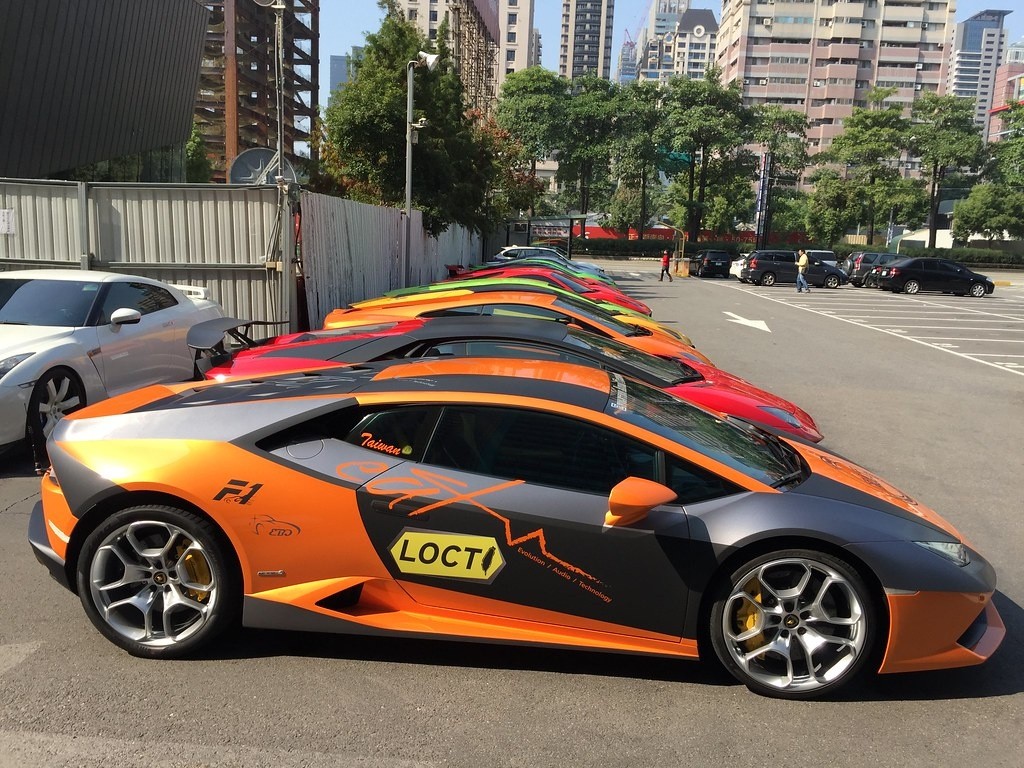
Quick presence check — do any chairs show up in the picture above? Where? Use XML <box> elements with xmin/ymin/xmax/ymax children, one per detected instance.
<box><xmin>410</xmin><ymin>407</ymin><xmax>498</xmax><ymax>476</ymax></box>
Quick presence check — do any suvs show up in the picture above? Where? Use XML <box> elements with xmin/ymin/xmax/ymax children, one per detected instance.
<box><xmin>842</xmin><ymin>252</ymin><xmax>913</xmax><ymax>289</ymax></box>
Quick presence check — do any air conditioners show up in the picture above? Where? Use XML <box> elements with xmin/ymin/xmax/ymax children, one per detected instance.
<box><xmin>742</xmin><ymin>0</ymin><xmax>944</xmax><ymax>171</ymax></box>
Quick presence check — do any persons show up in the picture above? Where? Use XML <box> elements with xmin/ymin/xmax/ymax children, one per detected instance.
<box><xmin>658</xmin><ymin>249</ymin><xmax>673</xmax><ymax>282</ymax></box>
<box><xmin>795</xmin><ymin>248</ymin><xmax>810</xmax><ymax>293</ymax></box>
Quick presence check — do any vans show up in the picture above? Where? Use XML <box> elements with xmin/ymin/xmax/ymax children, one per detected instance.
<box><xmin>729</xmin><ymin>248</ymin><xmax>848</xmax><ymax>288</ymax></box>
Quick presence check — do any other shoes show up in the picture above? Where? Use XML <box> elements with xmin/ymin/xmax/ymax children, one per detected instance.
<box><xmin>797</xmin><ymin>290</ymin><xmax>801</xmax><ymax>292</ymax></box>
<box><xmin>805</xmin><ymin>289</ymin><xmax>810</xmax><ymax>293</ymax></box>
<box><xmin>659</xmin><ymin>279</ymin><xmax>663</xmax><ymax>281</ymax></box>
<box><xmin>670</xmin><ymin>278</ymin><xmax>673</xmax><ymax>282</ymax></box>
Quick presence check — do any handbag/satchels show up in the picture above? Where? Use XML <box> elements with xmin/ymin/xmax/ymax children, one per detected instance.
<box><xmin>801</xmin><ymin>267</ymin><xmax>809</xmax><ymax>275</ymax></box>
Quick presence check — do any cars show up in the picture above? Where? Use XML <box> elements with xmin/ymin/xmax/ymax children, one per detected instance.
<box><xmin>1</xmin><ymin>269</ymin><xmax>227</xmax><ymax>469</ymax></box>
<box><xmin>690</xmin><ymin>248</ymin><xmax>731</xmax><ymax>277</ymax></box>
<box><xmin>870</xmin><ymin>258</ymin><xmax>991</xmax><ymax>297</ymax></box>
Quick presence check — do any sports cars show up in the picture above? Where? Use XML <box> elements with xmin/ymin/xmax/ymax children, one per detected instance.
<box><xmin>24</xmin><ymin>353</ymin><xmax>1005</xmax><ymax>696</ymax></box>
<box><xmin>205</xmin><ymin>241</ymin><xmax>824</xmax><ymax>447</ymax></box>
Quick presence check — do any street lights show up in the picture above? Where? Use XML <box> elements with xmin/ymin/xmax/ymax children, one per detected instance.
<box><xmin>407</xmin><ymin>51</ymin><xmax>441</xmax><ymax>297</ymax></box>
